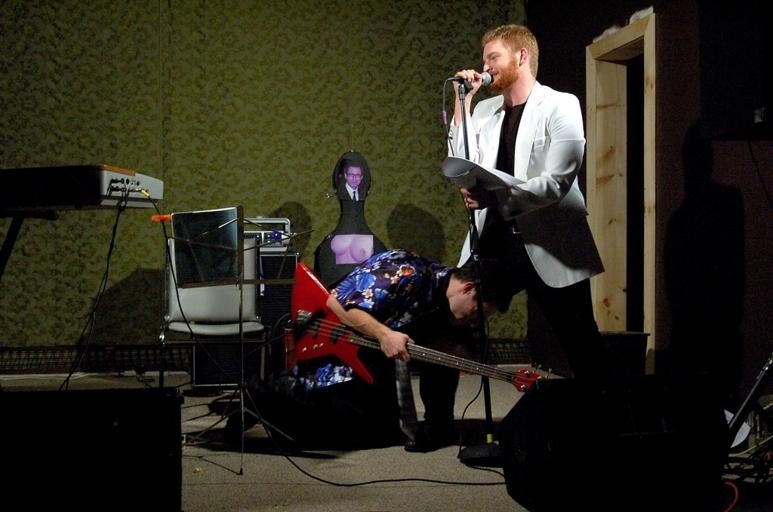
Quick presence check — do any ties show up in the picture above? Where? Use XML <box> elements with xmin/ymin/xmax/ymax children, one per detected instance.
<box><xmin>353</xmin><ymin>191</ymin><xmax>355</xmax><ymax>204</ymax></box>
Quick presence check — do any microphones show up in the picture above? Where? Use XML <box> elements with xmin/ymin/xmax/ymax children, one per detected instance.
<box><xmin>447</xmin><ymin>72</ymin><xmax>492</xmax><ymax>87</ymax></box>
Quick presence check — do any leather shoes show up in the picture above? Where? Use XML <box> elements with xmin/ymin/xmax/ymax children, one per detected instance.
<box><xmin>404</xmin><ymin>420</ymin><xmax>422</xmax><ymax>454</ymax></box>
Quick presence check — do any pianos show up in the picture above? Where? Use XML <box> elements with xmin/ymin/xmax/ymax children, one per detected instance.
<box><xmin>0</xmin><ymin>164</ymin><xmax>164</xmax><ymax>207</ymax></box>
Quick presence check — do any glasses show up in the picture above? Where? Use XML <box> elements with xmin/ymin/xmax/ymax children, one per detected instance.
<box><xmin>348</xmin><ymin>173</ymin><xmax>360</xmax><ymax>178</ymax></box>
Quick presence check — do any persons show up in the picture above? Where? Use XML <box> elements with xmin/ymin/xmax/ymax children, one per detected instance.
<box><xmin>405</xmin><ymin>23</ymin><xmax>606</xmax><ymax>453</ymax></box>
<box><xmin>337</xmin><ymin>161</ymin><xmax>367</xmax><ymax>201</ymax></box>
<box><xmin>328</xmin><ymin>247</ymin><xmax>510</xmax><ymax>452</ymax></box>
<box><xmin>330</xmin><ymin>234</ymin><xmax>374</xmax><ymax>264</ymax></box>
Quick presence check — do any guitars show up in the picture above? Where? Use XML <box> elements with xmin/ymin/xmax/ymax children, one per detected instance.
<box><xmin>283</xmin><ymin>261</ymin><xmax>546</xmax><ymax>392</ymax></box>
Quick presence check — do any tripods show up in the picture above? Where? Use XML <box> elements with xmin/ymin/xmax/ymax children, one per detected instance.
<box><xmin>182</xmin><ymin>283</ymin><xmax>295</xmax><ymax>475</ymax></box>
